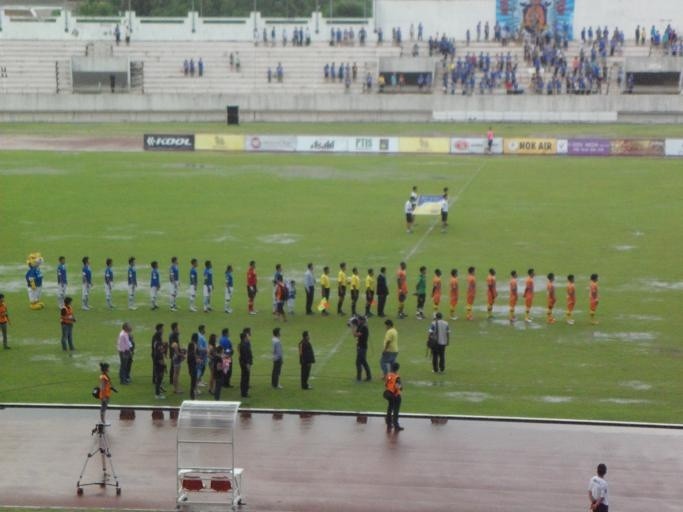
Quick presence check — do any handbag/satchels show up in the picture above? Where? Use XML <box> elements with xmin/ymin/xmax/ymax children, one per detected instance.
<box><xmin>383</xmin><ymin>390</ymin><xmax>395</xmax><ymax>401</ymax></box>
<box><xmin>426</xmin><ymin>338</ymin><xmax>438</xmax><ymax>350</ymax></box>
<box><xmin>92</xmin><ymin>387</ymin><xmax>100</xmax><ymax>398</ymax></box>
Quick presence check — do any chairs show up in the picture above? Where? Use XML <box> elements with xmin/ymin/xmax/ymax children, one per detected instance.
<box><xmin>210</xmin><ymin>477</ymin><xmax>232</xmax><ymax>491</ymax></box>
<box><xmin>182</xmin><ymin>475</ymin><xmax>205</xmax><ymax>491</ymax></box>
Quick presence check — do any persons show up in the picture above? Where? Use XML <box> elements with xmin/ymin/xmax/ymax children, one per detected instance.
<box><xmin>634</xmin><ymin>24</ymin><xmax>682</xmax><ymax>57</ymax></box>
<box><xmin>80</xmin><ymin>256</ymin><xmax>94</xmax><ymax>311</ymax></box>
<box><xmin>56</xmin><ymin>255</ymin><xmax>68</xmax><ymax>309</ymax></box>
<box><xmin>486</xmin><ymin>127</ymin><xmax>493</xmax><ymax>151</ymax></box>
<box><xmin>59</xmin><ymin>296</ymin><xmax>77</xmax><ymax>352</ymax></box>
<box><xmin>299</xmin><ymin>331</ymin><xmax>317</xmax><ymax>389</ymax></box>
<box><xmin>427</xmin><ymin>313</ymin><xmax>450</xmax><ymax>373</ymax></box>
<box><xmin>151</xmin><ymin>322</ymin><xmax>253</xmax><ymax>402</ymax></box>
<box><xmin>328</xmin><ymin>22</ymin><xmax>457</xmax><ymax>62</ymax></box>
<box><xmin>403</xmin><ymin>184</ymin><xmax>451</xmax><ymax>235</ymax></box>
<box><xmin>271</xmin><ymin>328</ymin><xmax>286</xmax><ymax>390</ymax></box>
<box><xmin>184</xmin><ymin>57</ymin><xmax>205</xmax><ymax>77</ymax></box>
<box><xmin>378</xmin><ymin>320</ymin><xmax>398</xmax><ymax>378</ymax></box>
<box><xmin>0</xmin><ymin>293</ymin><xmax>12</xmax><ymax>349</ymax></box>
<box><xmin>323</xmin><ymin>61</ymin><xmax>449</xmax><ymax>93</ymax></box>
<box><xmin>396</xmin><ymin>262</ymin><xmax>459</xmax><ymax>321</ymax></box>
<box><xmin>383</xmin><ymin>362</ymin><xmax>405</xmax><ymax>432</ymax></box>
<box><xmin>98</xmin><ymin>363</ymin><xmax>112</xmax><ymax>426</ymax></box>
<box><xmin>545</xmin><ymin>272</ymin><xmax>557</xmax><ymax>323</ymax></box>
<box><xmin>465</xmin><ymin>267</ymin><xmax>518</xmax><ymax>321</ymax></box>
<box><xmin>522</xmin><ymin>269</ymin><xmax>535</xmax><ymax>323</ymax></box>
<box><xmin>268</xmin><ymin>63</ymin><xmax>283</xmax><ymax>83</ymax></box>
<box><xmin>589</xmin><ymin>274</ymin><xmax>600</xmax><ymax>326</ymax></box>
<box><xmin>228</xmin><ymin>52</ymin><xmax>242</xmax><ymax>72</ymax></box>
<box><xmin>104</xmin><ymin>255</ymin><xmax>389</xmax><ymax>321</ymax></box>
<box><xmin>114</xmin><ymin>23</ymin><xmax>133</xmax><ymax>47</ymax></box>
<box><xmin>262</xmin><ymin>24</ymin><xmax>312</xmax><ymax>48</ymax></box>
<box><xmin>442</xmin><ymin>20</ymin><xmax>633</xmax><ymax>94</ymax></box>
<box><xmin>566</xmin><ymin>274</ymin><xmax>577</xmax><ymax>325</ymax></box>
<box><xmin>118</xmin><ymin>323</ymin><xmax>138</xmax><ymax>384</ymax></box>
<box><xmin>353</xmin><ymin>316</ymin><xmax>372</xmax><ymax>385</ymax></box>
<box><xmin>587</xmin><ymin>463</ymin><xmax>608</xmax><ymax>512</ymax></box>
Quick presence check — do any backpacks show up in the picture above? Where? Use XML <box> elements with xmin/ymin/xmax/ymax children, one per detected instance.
<box><xmin>276</xmin><ymin>283</ymin><xmax>288</xmax><ymax>301</ymax></box>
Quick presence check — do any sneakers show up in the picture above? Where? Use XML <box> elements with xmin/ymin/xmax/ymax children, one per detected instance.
<box><xmin>357</xmin><ymin>377</ymin><xmax>387</xmax><ymax>384</ymax></box>
<box><xmin>63</xmin><ymin>349</ymin><xmax>75</xmax><ymax>353</ymax></box>
<box><xmin>406</xmin><ymin>223</ymin><xmax>449</xmax><ymax>235</ymax></box>
<box><xmin>3</xmin><ymin>346</ymin><xmax>11</xmax><ymax>350</ymax></box>
<box><xmin>432</xmin><ymin>368</ymin><xmax>446</xmax><ymax>374</ymax></box>
<box><xmin>100</xmin><ymin>423</ymin><xmax>111</xmax><ymax>427</ymax></box>
<box><xmin>386</xmin><ymin>423</ymin><xmax>404</xmax><ymax>431</ymax></box>
<box><xmin>119</xmin><ymin>377</ymin><xmax>314</xmax><ymax>401</ymax></box>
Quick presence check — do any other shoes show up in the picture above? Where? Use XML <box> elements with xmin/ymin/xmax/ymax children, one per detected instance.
<box><xmin>170</xmin><ymin>305</ymin><xmax>294</xmax><ymax>316</ymax></box>
<box><xmin>304</xmin><ymin>309</ymin><xmax>438</xmax><ymax>319</ymax></box>
<box><xmin>57</xmin><ymin>304</ymin><xmax>161</xmax><ymax>311</ymax></box>
<box><xmin>449</xmin><ymin>314</ymin><xmax>598</xmax><ymax>324</ymax></box>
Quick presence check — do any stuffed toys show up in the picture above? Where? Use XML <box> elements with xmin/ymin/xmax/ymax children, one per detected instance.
<box><xmin>24</xmin><ymin>251</ymin><xmax>45</xmax><ymax>309</ymax></box>
